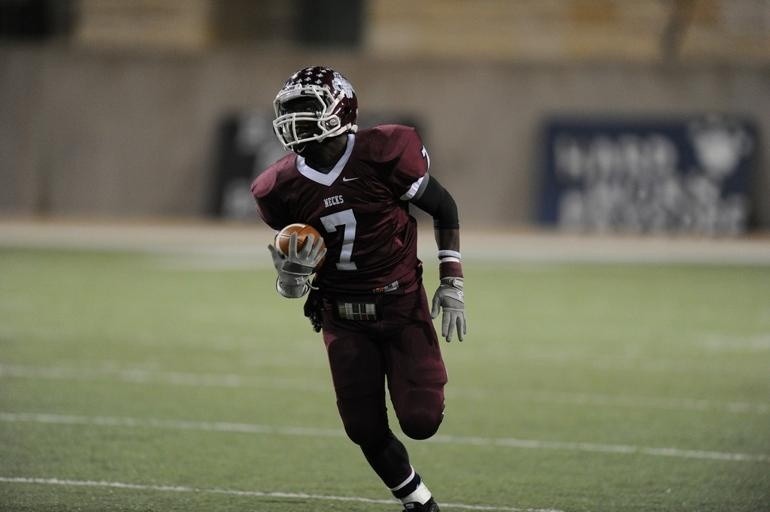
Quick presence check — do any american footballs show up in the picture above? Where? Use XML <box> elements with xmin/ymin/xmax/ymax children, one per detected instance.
<box><xmin>274</xmin><ymin>223</ymin><xmax>327</xmax><ymax>274</ymax></box>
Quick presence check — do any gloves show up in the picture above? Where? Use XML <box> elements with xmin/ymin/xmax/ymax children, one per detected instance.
<box><xmin>268</xmin><ymin>233</ymin><xmax>327</xmax><ymax>297</ymax></box>
<box><xmin>430</xmin><ymin>276</ymin><xmax>467</xmax><ymax>341</ymax></box>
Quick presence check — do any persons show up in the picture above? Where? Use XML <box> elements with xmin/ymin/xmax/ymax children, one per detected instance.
<box><xmin>248</xmin><ymin>64</ymin><xmax>468</xmax><ymax>512</ymax></box>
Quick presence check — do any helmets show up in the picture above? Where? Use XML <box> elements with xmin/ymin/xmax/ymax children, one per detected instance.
<box><xmin>271</xmin><ymin>66</ymin><xmax>358</xmax><ymax>152</ymax></box>
